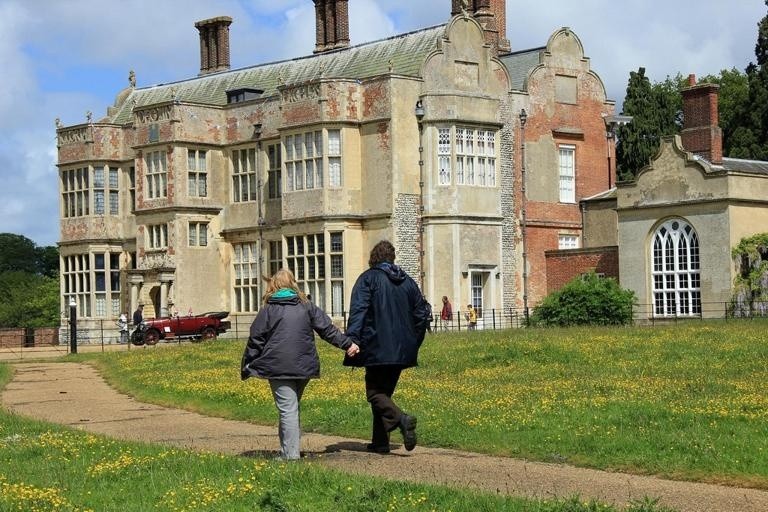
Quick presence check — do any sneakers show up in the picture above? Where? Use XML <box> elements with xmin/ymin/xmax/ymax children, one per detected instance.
<box><xmin>367</xmin><ymin>444</ymin><xmax>390</xmax><ymax>453</ymax></box>
<box><xmin>399</xmin><ymin>414</ymin><xmax>417</xmax><ymax>451</ymax></box>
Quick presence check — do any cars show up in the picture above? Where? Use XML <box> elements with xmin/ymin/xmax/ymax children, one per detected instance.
<box><xmin>132</xmin><ymin>312</ymin><xmax>231</xmax><ymax>345</ymax></box>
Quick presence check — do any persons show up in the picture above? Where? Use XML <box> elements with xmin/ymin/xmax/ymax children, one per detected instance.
<box><xmin>165</xmin><ymin>299</ymin><xmax>178</xmax><ymax>320</ymax></box>
<box><xmin>117</xmin><ymin>309</ymin><xmax>131</xmax><ymax>344</ymax></box>
<box><xmin>134</xmin><ymin>303</ymin><xmax>146</xmax><ymax>330</ymax></box>
<box><xmin>465</xmin><ymin>303</ymin><xmax>477</xmax><ymax>330</ymax></box>
<box><xmin>240</xmin><ymin>270</ymin><xmax>359</xmax><ymax>460</ymax></box>
<box><xmin>346</xmin><ymin>242</ymin><xmax>428</xmax><ymax>455</ymax></box>
<box><xmin>422</xmin><ymin>297</ymin><xmax>434</xmax><ymax>333</ymax></box>
<box><xmin>441</xmin><ymin>295</ymin><xmax>453</xmax><ymax>333</ymax></box>
